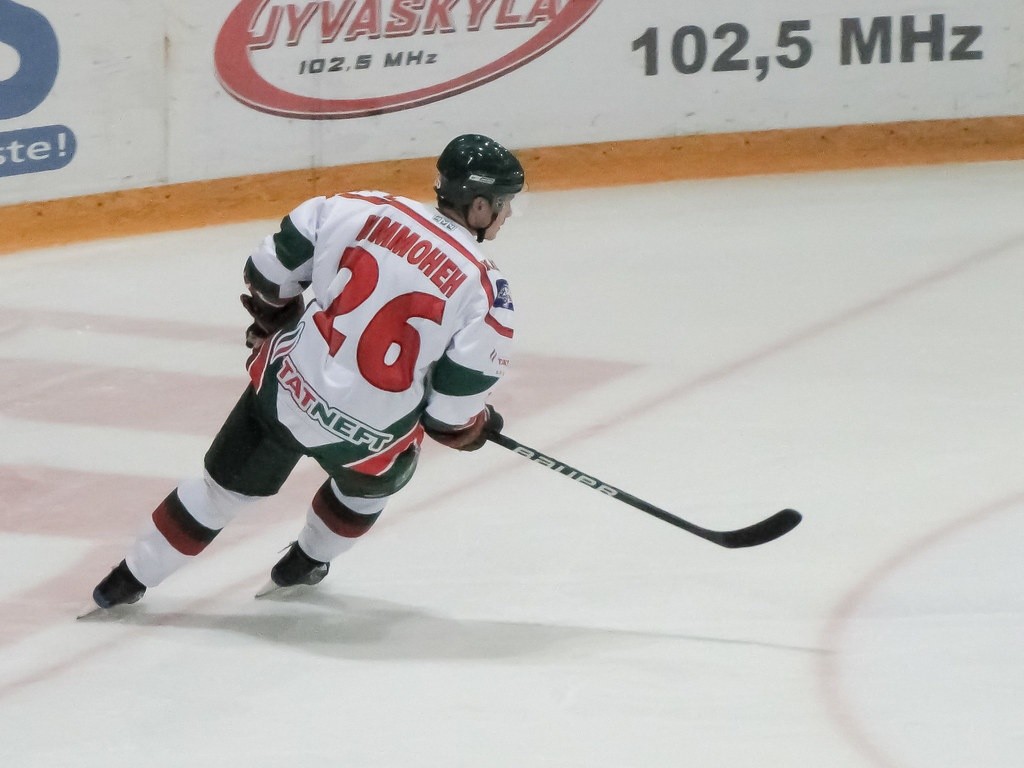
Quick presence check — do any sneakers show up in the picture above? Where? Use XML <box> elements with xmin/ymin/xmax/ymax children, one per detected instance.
<box><xmin>256</xmin><ymin>540</ymin><xmax>329</xmax><ymax>600</ymax></box>
<box><xmin>77</xmin><ymin>559</ymin><xmax>145</xmax><ymax>617</ymax></box>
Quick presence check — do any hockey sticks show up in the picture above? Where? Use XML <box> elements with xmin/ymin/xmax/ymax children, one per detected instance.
<box><xmin>485</xmin><ymin>428</ymin><xmax>804</xmax><ymax>550</ymax></box>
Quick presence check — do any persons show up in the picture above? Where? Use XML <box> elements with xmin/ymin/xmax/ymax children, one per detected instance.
<box><xmin>75</xmin><ymin>135</ymin><xmax>527</xmax><ymax>618</ymax></box>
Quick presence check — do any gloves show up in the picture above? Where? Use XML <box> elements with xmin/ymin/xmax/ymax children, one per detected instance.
<box><xmin>429</xmin><ymin>402</ymin><xmax>503</xmax><ymax>453</ymax></box>
<box><xmin>241</xmin><ymin>296</ymin><xmax>304</xmax><ymax>348</ymax></box>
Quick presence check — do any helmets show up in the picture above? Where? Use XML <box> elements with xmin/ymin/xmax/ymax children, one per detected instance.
<box><xmin>434</xmin><ymin>133</ymin><xmax>524</xmax><ymax>206</ymax></box>
